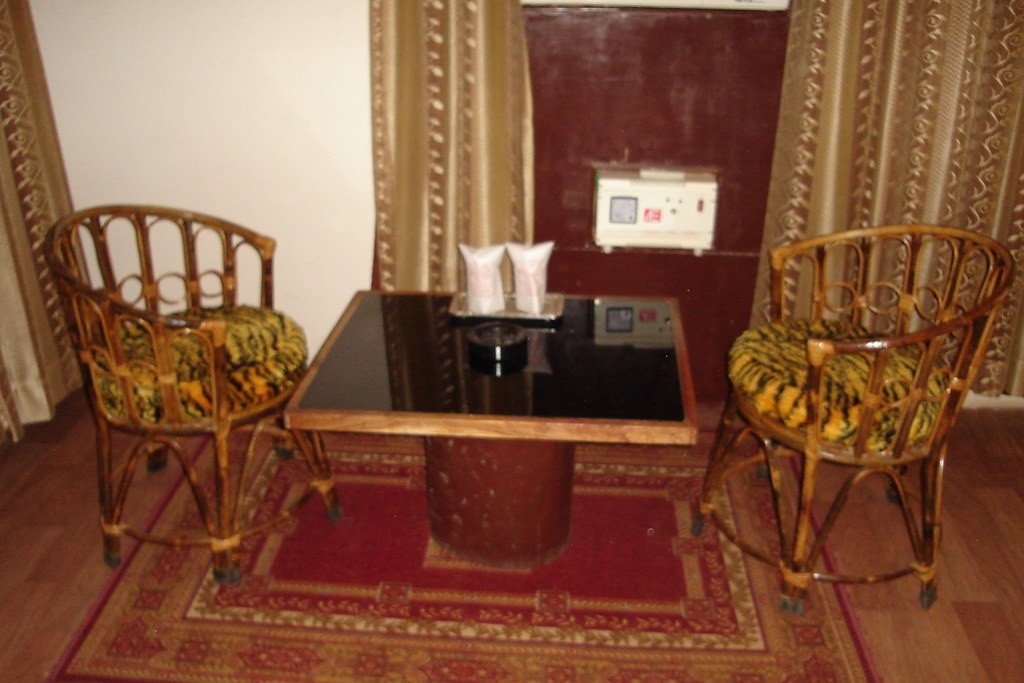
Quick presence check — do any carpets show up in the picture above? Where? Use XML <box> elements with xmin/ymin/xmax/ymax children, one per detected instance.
<box><xmin>52</xmin><ymin>427</ymin><xmax>884</xmax><ymax>683</ymax></box>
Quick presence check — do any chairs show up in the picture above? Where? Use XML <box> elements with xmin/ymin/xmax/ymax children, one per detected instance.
<box><xmin>46</xmin><ymin>205</ymin><xmax>343</xmax><ymax>588</ymax></box>
<box><xmin>693</xmin><ymin>225</ymin><xmax>1016</xmax><ymax>610</ymax></box>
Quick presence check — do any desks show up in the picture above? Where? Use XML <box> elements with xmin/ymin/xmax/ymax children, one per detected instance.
<box><xmin>282</xmin><ymin>290</ymin><xmax>697</xmax><ymax>567</ymax></box>
